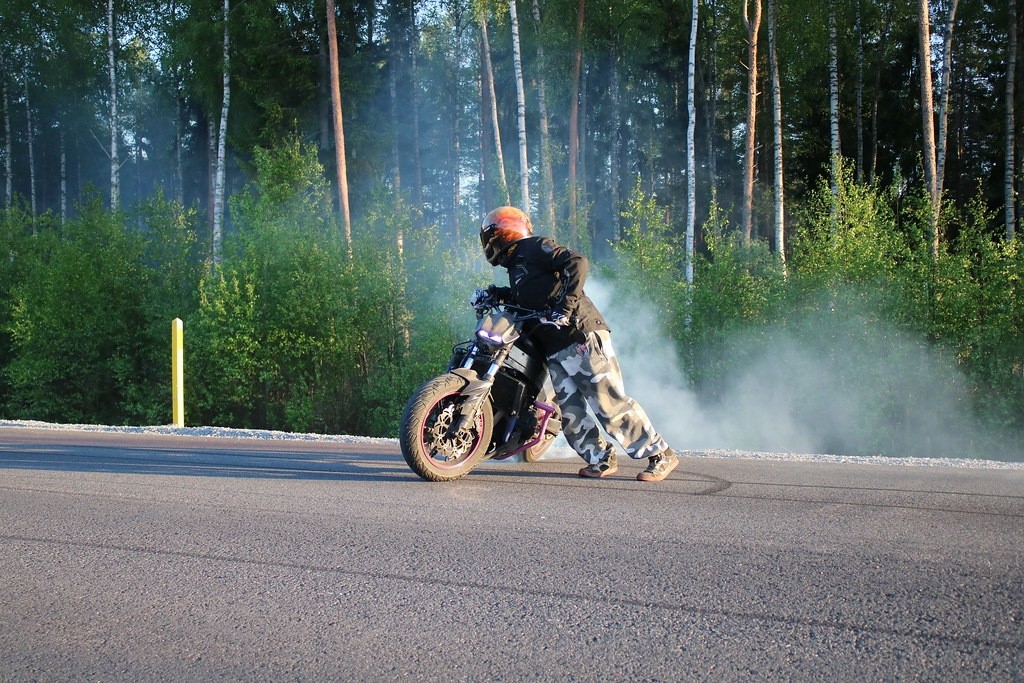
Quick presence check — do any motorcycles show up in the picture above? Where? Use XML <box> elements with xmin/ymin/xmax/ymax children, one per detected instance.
<box><xmin>399</xmin><ymin>290</ymin><xmax>579</xmax><ymax>482</ymax></box>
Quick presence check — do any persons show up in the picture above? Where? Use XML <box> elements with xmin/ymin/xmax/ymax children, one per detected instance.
<box><xmin>480</xmin><ymin>206</ymin><xmax>680</xmax><ymax>481</ymax></box>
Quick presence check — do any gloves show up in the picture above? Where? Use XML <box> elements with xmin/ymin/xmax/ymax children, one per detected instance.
<box><xmin>470</xmin><ymin>290</ymin><xmax>493</xmax><ymax>308</ymax></box>
<box><xmin>551</xmin><ymin>311</ymin><xmax>571</xmax><ymax>326</ymax></box>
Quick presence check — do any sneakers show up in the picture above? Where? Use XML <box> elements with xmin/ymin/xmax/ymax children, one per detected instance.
<box><xmin>637</xmin><ymin>447</ymin><xmax>680</xmax><ymax>481</ymax></box>
<box><xmin>579</xmin><ymin>441</ymin><xmax>618</xmax><ymax>478</ymax></box>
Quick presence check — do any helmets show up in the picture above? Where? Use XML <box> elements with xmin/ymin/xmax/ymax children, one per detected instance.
<box><xmin>480</xmin><ymin>206</ymin><xmax>534</xmax><ymax>267</ymax></box>
